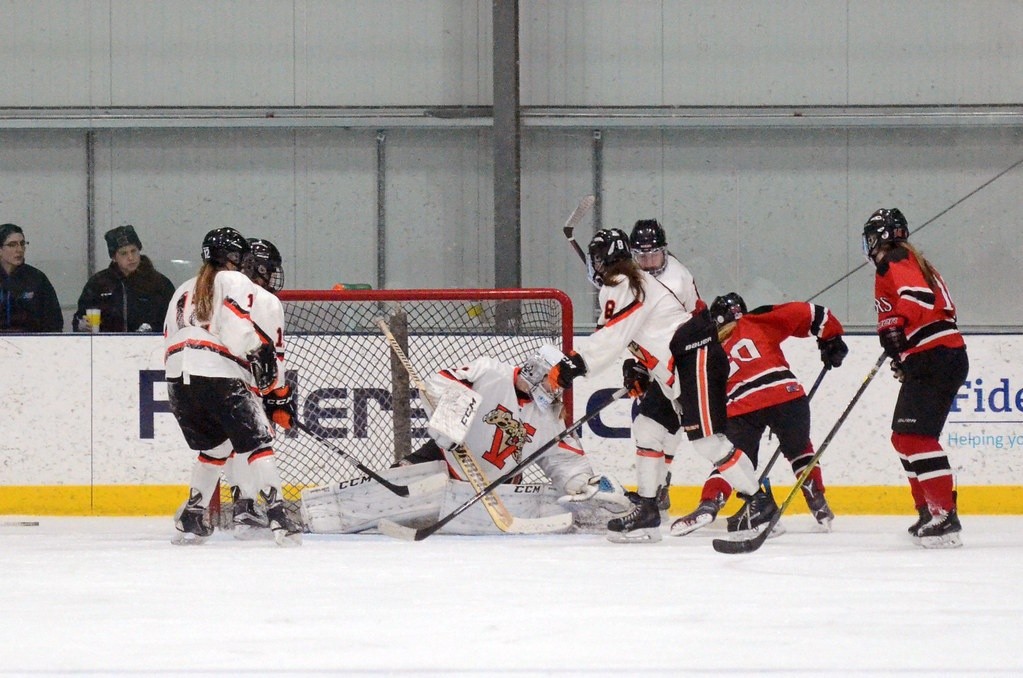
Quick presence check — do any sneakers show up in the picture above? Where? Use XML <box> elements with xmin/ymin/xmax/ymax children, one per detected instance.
<box><xmin>917</xmin><ymin>490</ymin><xmax>962</xmax><ymax>549</ymax></box>
<box><xmin>228</xmin><ymin>486</ymin><xmax>276</xmax><ymax>541</ymax></box>
<box><xmin>802</xmin><ymin>480</ymin><xmax>833</xmax><ymax>533</ymax></box>
<box><xmin>261</xmin><ymin>488</ymin><xmax>302</xmax><ymax>547</ymax></box>
<box><xmin>729</xmin><ymin>479</ymin><xmax>788</xmax><ymax>543</ymax></box>
<box><xmin>623</xmin><ymin>474</ymin><xmax>671</xmax><ymax>518</ymax></box>
<box><xmin>172</xmin><ymin>488</ymin><xmax>214</xmax><ymax>545</ymax></box>
<box><xmin>668</xmin><ymin>493</ymin><xmax>726</xmax><ymax>537</ymax></box>
<box><xmin>909</xmin><ymin>501</ymin><xmax>934</xmax><ymax>545</ymax></box>
<box><xmin>606</xmin><ymin>485</ymin><xmax>662</xmax><ymax>542</ymax></box>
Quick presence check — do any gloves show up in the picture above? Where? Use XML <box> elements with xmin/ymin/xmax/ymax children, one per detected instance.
<box><xmin>549</xmin><ymin>351</ymin><xmax>587</xmax><ymax>392</ymax></box>
<box><xmin>817</xmin><ymin>334</ymin><xmax>851</xmax><ymax>368</ymax></box>
<box><xmin>265</xmin><ymin>387</ymin><xmax>294</xmax><ymax>432</ymax></box>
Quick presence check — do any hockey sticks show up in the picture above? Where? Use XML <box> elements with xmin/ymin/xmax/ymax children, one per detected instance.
<box><xmin>294</xmin><ymin>420</ymin><xmax>449</xmax><ymax>498</ymax></box>
<box><xmin>375</xmin><ymin>386</ymin><xmax>631</xmax><ymax>541</ymax></box>
<box><xmin>711</xmin><ymin>350</ymin><xmax>887</xmax><ymax>554</ymax></box>
<box><xmin>374</xmin><ymin>315</ymin><xmax>576</xmax><ymax>534</ymax></box>
<box><xmin>724</xmin><ymin>365</ymin><xmax>828</xmax><ymax>523</ymax></box>
<box><xmin>561</xmin><ymin>192</ymin><xmax>597</xmax><ymax>266</ymax></box>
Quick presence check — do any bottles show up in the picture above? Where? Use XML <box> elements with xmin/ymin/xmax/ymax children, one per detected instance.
<box><xmin>332</xmin><ymin>283</ymin><xmax>372</xmax><ymax>290</ymax></box>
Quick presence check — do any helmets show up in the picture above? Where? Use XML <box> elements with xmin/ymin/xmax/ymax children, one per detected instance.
<box><xmin>201</xmin><ymin>228</ymin><xmax>251</xmax><ymax>262</ymax></box>
<box><xmin>707</xmin><ymin>292</ymin><xmax>747</xmax><ymax>331</ymax></box>
<box><xmin>630</xmin><ymin>218</ymin><xmax>667</xmax><ymax>276</ymax></box>
<box><xmin>514</xmin><ymin>343</ymin><xmax>576</xmax><ymax>412</ymax></box>
<box><xmin>244</xmin><ymin>237</ymin><xmax>281</xmax><ymax>268</ymax></box>
<box><xmin>861</xmin><ymin>207</ymin><xmax>908</xmax><ymax>270</ymax></box>
<box><xmin>588</xmin><ymin>229</ymin><xmax>630</xmax><ymax>289</ymax></box>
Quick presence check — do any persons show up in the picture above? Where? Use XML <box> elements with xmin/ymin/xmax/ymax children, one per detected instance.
<box><xmin>620</xmin><ymin>219</ymin><xmax>710</xmax><ymax>524</ymax></box>
<box><xmin>670</xmin><ymin>293</ymin><xmax>848</xmax><ymax>531</ymax></box>
<box><xmin>548</xmin><ymin>228</ymin><xmax>785</xmax><ymax>544</ymax></box>
<box><xmin>273</xmin><ymin>345</ymin><xmax>634</xmax><ymax>534</ymax></box>
<box><xmin>161</xmin><ymin>228</ymin><xmax>302</xmax><ymax>549</ymax></box>
<box><xmin>0</xmin><ymin>223</ymin><xmax>64</xmax><ymax>333</ymax></box>
<box><xmin>862</xmin><ymin>206</ymin><xmax>969</xmax><ymax>550</ymax></box>
<box><xmin>71</xmin><ymin>225</ymin><xmax>177</xmax><ymax>334</ymax></box>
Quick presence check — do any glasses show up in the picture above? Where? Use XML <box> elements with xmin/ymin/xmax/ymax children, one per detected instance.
<box><xmin>0</xmin><ymin>239</ymin><xmax>30</xmax><ymax>248</ymax></box>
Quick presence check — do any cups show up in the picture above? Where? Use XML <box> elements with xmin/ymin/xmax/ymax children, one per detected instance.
<box><xmin>86</xmin><ymin>309</ymin><xmax>101</xmax><ymax>332</ymax></box>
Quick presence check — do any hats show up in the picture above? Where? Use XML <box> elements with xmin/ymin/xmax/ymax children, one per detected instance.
<box><xmin>104</xmin><ymin>225</ymin><xmax>143</xmax><ymax>258</ymax></box>
